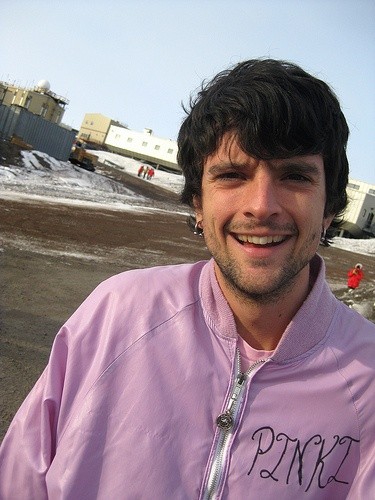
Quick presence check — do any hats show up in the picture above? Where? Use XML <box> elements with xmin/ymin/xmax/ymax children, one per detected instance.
<box><xmin>355</xmin><ymin>263</ymin><xmax>362</xmax><ymax>270</ymax></box>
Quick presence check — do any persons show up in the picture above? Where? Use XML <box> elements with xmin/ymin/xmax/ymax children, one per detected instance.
<box><xmin>137</xmin><ymin>165</ymin><xmax>154</xmax><ymax>180</ymax></box>
<box><xmin>0</xmin><ymin>59</ymin><xmax>375</xmax><ymax>500</ymax></box>
<box><xmin>348</xmin><ymin>263</ymin><xmax>362</xmax><ymax>290</ymax></box>
